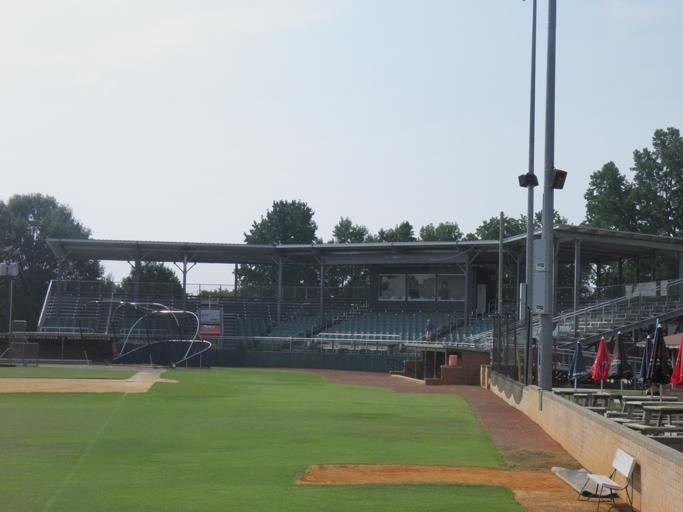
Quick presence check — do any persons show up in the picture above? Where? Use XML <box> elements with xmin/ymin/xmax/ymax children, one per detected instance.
<box><xmin>424</xmin><ymin>319</ymin><xmax>435</xmax><ymax>340</ymax></box>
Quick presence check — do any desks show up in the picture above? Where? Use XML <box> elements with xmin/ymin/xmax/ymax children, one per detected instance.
<box><xmin>551</xmin><ymin>388</ymin><xmax>683</xmax><ymax>419</ymax></box>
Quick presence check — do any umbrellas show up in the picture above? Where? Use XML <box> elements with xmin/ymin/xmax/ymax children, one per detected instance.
<box><xmin>607</xmin><ymin>332</ymin><xmax>633</xmax><ymax>390</ymax></box>
<box><xmin>566</xmin><ymin>340</ymin><xmax>588</xmax><ymax>389</ymax></box>
<box><xmin>646</xmin><ymin>318</ymin><xmax>673</xmax><ymax>402</ymax></box>
<box><xmin>591</xmin><ymin>336</ymin><xmax>611</xmax><ymax>392</ymax></box>
<box><xmin>640</xmin><ymin>335</ymin><xmax>655</xmax><ymax>396</ymax></box>
<box><xmin>670</xmin><ymin>335</ymin><xmax>683</xmax><ymax>390</ymax></box>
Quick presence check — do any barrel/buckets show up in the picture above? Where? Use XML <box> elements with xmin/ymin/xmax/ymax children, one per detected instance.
<box><xmin>449</xmin><ymin>355</ymin><xmax>457</xmax><ymax>368</ymax></box>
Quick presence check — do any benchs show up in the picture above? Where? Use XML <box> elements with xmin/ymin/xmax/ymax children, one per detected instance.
<box><xmin>584</xmin><ymin>406</ymin><xmax>683</xmax><ymax>444</ymax></box>
<box><xmin>577</xmin><ymin>448</ymin><xmax>637</xmax><ymax>511</ymax></box>
